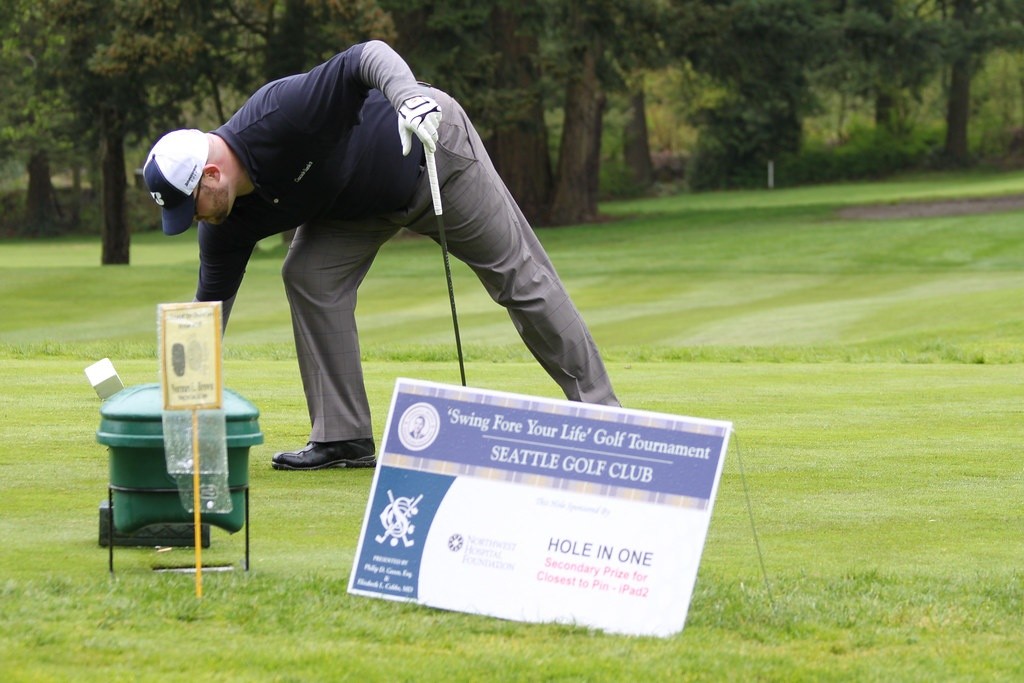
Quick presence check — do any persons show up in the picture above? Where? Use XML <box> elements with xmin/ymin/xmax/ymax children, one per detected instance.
<box><xmin>143</xmin><ymin>39</ymin><xmax>624</xmax><ymax>470</ymax></box>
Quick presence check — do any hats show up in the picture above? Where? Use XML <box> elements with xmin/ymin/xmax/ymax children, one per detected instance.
<box><xmin>142</xmin><ymin>128</ymin><xmax>211</xmax><ymax>236</ymax></box>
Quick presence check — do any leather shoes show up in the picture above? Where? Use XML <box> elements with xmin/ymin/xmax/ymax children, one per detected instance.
<box><xmin>270</xmin><ymin>438</ymin><xmax>376</xmax><ymax>470</ymax></box>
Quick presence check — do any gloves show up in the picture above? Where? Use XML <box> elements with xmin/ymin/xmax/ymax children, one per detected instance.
<box><xmin>396</xmin><ymin>96</ymin><xmax>442</xmax><ymax>157</ymax></box>
